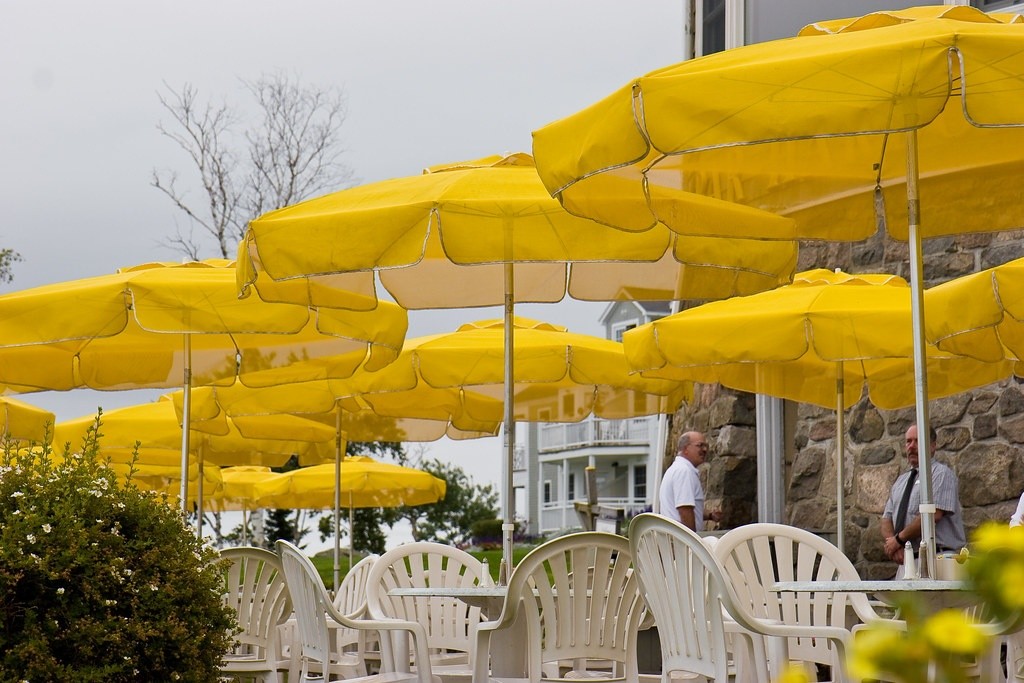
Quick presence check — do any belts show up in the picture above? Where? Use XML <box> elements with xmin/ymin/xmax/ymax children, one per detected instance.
<box><xmin>914</xmin><ymin>546</ymin><xmax>955</xmax><ymax>560</ymax></box>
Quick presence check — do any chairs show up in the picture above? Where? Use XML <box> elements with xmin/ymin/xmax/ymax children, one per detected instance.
<box><xmin>210</xmin><ymin>513</ymin><xmax>1023</xmax><ymax>682</ymax></box>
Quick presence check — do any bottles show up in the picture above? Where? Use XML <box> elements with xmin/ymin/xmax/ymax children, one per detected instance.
<box><xmin>901</xmin><ymin>540</ymin><xmax>932</xmax><ymax>581</ymax></box>
<box><xmin>497</xmin><ymin>558</ymin><xmax>507</xmax><ymax>586</ymax></box>
<box><xmin>481</xmin><ymin>557</ymin><xmax>490</xmax><ymax>588</ymax></box>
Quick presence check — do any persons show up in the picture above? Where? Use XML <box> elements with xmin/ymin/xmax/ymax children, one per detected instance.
<box><xmin>881</xmin><ymin>425</ymin><xmax>965</xmax><ymax>580</ymax></box>
<box><xmin>660</xmin><ymin>432</ymin><xmax>708</xmax><ymax>533</ymax></box>
<box><xmin>1009</xmin><ymin>492</ymin><xmax>1024</xmax><ymax>529</ymax></box>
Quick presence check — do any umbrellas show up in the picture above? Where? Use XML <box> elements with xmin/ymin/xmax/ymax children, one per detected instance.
<box><xmin>0</xmin><ymin>257</ymin><xmax>409</xmax><ymax>518</ymax></box>
<box><xmin>172</xmin><ymin>356</ymin><xmax>504</xmax><ymax>596</ymax></box>
<box><xmin>255</xmin><ymin>456</ymin><xmax>446</xmax><ymax>568</ymax></box>
<box><xmin>923</xmin><ymin>256</ymin><xmax>1023</xmax><ymax>380</ymax></box>
<box><xmin>622</xmin><ymin>268</ymin><xmax>1015</xmax><ymax>552</ymax></box>
<box><xmin>327</xmin><ymin>316</ymin><xmax>695</xmax><ymax>423</ymax></box>
<box><xmin>0</xmin><ymin>398</ymin><xmax>55</xmax><ymax>450</ymax></box>
<box><xmin>0</xmin><ymin>394</ymin><xmax>349</xmax><ymax>548</ymax></box>
<box><xmin>235</xmin><ymin>150</ymin><xmax>802</xmax><ymax>583</ymax></box>
<box><xmin>535</xmin><ymin>7</ymin><xmax>1024</xmax><ymax>574</ymax></box>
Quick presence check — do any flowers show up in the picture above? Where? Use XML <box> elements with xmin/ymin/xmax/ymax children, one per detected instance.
<box><xmin>774</xmin><ymin>521</ymin><xmax>1023</xmax><ymax>683</ymax></box>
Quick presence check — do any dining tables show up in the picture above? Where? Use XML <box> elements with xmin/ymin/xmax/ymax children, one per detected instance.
<box><xmin>389</xmin><ymin>588</ymin><xmax>623</xmax><ymax>677</ymax></box>
<box><xmin>769</xmin><ymin>581</ymin><xmax>989</xmax><ymax>616</ymax></box>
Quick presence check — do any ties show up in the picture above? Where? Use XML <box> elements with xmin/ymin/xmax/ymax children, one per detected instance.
<box><xmin>894</xmin><ymin>468</ymin><xmax>917</xmax><ymax>535</ymax></box>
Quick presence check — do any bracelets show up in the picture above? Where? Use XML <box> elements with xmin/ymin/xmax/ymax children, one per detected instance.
<box><xmin>894</xmin><ymin>532</ymin><xmax>905</xmax><ymax>547</ymax></box>
